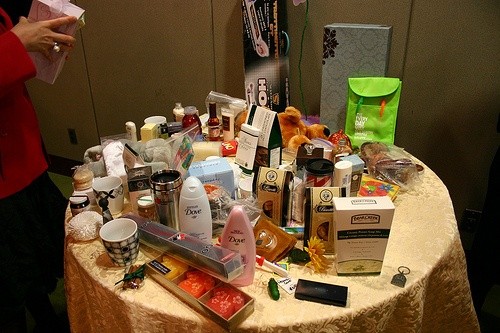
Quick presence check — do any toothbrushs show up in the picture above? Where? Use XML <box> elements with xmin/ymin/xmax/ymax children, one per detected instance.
<box><xmin>218</xmin><ymin>236</ymin><xmax>289</xmax><ymax>278</ymax></box>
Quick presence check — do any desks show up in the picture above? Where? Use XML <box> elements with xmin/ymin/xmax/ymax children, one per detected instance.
<box><xmin>64</xmin><ymin>146</ymin><xmax>480</xmax><ymax>333</ymax></box>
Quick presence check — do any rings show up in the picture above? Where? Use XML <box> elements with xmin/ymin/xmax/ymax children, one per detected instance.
<box><xmin>52</xmin><ymin>42</ymin><xmax>61</xmax><ymax>52</ymax></box>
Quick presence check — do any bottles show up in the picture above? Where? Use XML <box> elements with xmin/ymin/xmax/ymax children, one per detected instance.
<box><xmin>138</xmin><ymin>196</ymin><xmax>159</xmax><ymax>224</ymax></box>
<box><xmin>333</xmin><ymin>161</ymin><xmax>352</xmax><ymax>197</ymax></box>
<box><xmin>182</xmin><ymin>105</ymin><xmax>202</xmax><ymax>138</ymax></box>
<box><xmin>172</xmin><ymin>102</ymin><xmax>185</xmax><ymax>122</ymax></box>
<box><xmin>208</xmin><ymin>101</ymin><xmax>221</xmax><ymax>142</ymax></box>
<box><xmin>69</xmin><ymin>193</ymin><xmax>92</xmax><ymax>217</ymax></box>
<box><xmin>73</xmin><ymin>171</ymin><xmax>97</xmax><ymax>208</ymax></box>
<box><xmin>305</xmin><ymin>158</ymin><xmax>334</xmax><ymax>187</ymax></box>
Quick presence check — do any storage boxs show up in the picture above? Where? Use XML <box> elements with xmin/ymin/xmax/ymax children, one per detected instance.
<box><xmin>117</xmin><ymin>0</ymin><xmax>402</xmax><ymax>332</ymax></box>
<box><xmin>26</xmin><ymin>0</ymin><xmax>86</xmax><ymax>85</ymax></box>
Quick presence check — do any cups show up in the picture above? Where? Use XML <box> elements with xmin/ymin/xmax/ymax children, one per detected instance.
<box><xmin>238</xmin><ymin>177</ymin><xmax>255</xmax><ymax>199</ymax></box>
<box><xmin>100</xmin><ymin>218</ymin><xmax>140</xmax><ymax>267</ymax></box>
<box><xmin>149</xmin><ymin>170</ymin><xmax>182</xmax><ymax>230</ymax></box>
<box><xmin>92</xmin><ymin>176</ymin><xmax>124</xmax><ymax>215</ymax></box>
<box><xmin>158</xmin><ymin>123</ymin><xmax>170</xmax><ymax>140</ymax></box>
<box><xmin>144</xmin><ymin>115</ymin><xmax>167</xmax><ymax>138</ymax></box>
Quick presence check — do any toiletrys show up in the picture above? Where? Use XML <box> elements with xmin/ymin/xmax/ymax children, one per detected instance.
<box><xmin>172</xmin><ymin>102</ymin><xmax>185</xmax><ymax>122</ymax></box>
<box><xmin>221</xmin><ymin>106</ymin><xmax>235</xmax><ymax>141</ymax></box>
<box><xmin>220</xmin><ymin>204</ymin><xmax>256</xmax><ymax>287</ymax></box>
<box><xmin>208</xmin><ymin>102</ymin><xmax>221</xmax><ymax>142</ymax></box>
<box><xmin>334</xmin><ymin>160</ymin><xmax>353</xmax><ymax>198</ymax></box>
<box><xmin>125</xmin><ymin>121</ymin><xmax>137</xmax><ymax>142</ymax></box>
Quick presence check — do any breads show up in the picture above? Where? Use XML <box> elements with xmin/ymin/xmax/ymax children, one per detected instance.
<box><xmin>73</xmin><ymin>170</ymin><xmax>94</xmax><ymax>190</ymax></box>
<box><xmin>68</xmin><ymin>211</ymin><xmax>103</xmax><ymax>241</ymax></box>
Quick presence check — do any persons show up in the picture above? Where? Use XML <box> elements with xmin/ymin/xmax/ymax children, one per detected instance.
<box><xmin>0</xmin><ymin>6</ymin><xmax>76</xmax><ymax>333</ymax></box>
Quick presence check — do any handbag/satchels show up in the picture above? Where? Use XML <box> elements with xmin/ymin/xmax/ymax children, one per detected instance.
<box><xmin>345</xmin><ymin>76</ymin><xmax>403</xmax><ymax>147</ymax></box>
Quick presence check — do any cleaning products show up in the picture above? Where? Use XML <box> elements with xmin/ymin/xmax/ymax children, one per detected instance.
<box><xmin>178</xmin><ymin>175</ymin><xmax>212</xmax><ymax>244</ymax></box>
